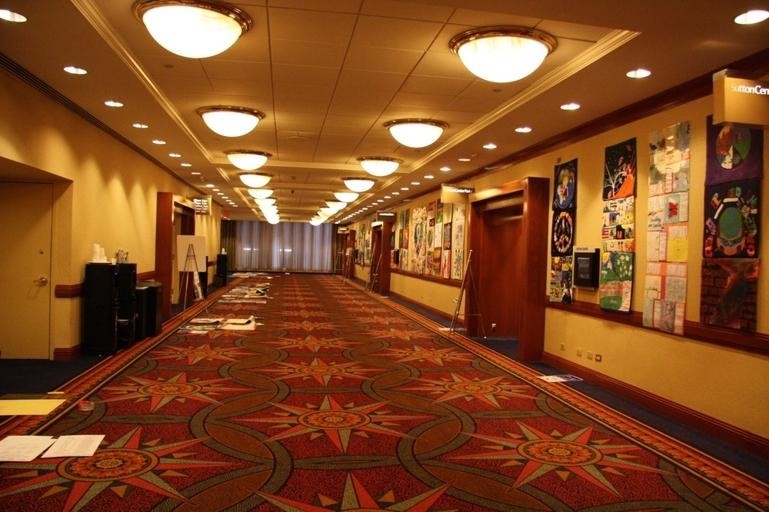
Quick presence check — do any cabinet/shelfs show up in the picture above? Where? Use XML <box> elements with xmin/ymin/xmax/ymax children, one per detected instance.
<box><xmin>80</xmin><ymin>262</ymin><xmax>163</xmax><ymax>356</ymax></box>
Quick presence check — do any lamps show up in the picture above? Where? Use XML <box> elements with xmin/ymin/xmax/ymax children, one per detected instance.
<box><xmin>446</xmin><ymin>23</ymin><xmax>560</xmax><ymax>84</ymax></box>
<box><xmin>195</xmin><ymin>103</ymin><xmax>275</xmax><ymax>190</ymax></box>
<box><xmin>326</xmin><ymin>118</ymin><xmax>450</xmax><ymax>211</ymax></box>
<box><xmin>132</xmin><ymin>0</ymin><xmax>254</xmax><ymax>60</ymax></box>
